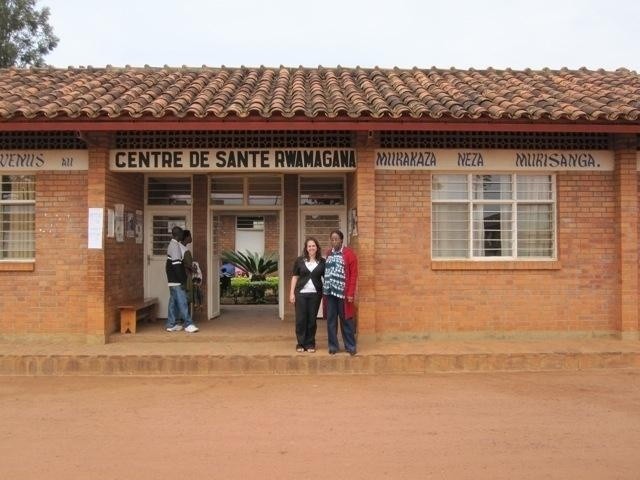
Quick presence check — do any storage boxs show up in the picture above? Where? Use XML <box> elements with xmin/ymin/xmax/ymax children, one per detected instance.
<box><xmin>116</xmin><ymin>297</ymin><xmax>157</xmax><ymax>335</ymax></box>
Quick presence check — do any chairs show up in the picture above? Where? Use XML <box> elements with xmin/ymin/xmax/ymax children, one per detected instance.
<box><xmin>328</xmin><ymin>350</ymin><xmax>356</xmax><ymax>355</ymax></box>
<box><xmin>296</xmin><ymin>347</ymin><xmax>316</xmax><ymax>353</ymax></box>
<box><xmin>185</xmin><ymin>324</ymin><xmax>198</xmax><ymax>333</ymax></box>
<box><xmin>166</xmin><ymin>324</ymin><xmax>182</xmax><ymax>332</ymax></box>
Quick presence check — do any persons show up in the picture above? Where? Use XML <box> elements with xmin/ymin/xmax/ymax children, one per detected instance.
<box><xmin>181</xmin><ymin>228</ymin><xmax>198</xmax><ymax>324</ymax></box>
<box><xmin>164</xmin><ymin>225</ymin><xmax>203</xmax><ymax>335</ymax></box>
<box><xmin>325</xmin><ymin>229</ymin><xmax>359</xmax><ymax>356</ymax></box>
<box><xmin>288</xmin><ymin>238</ymin><xmax>328</xmax><ymax>353</ymax></box>
<box><xmin>222</xmin><ymin>262</ymin><xmax>235</xmax><ymax>282</ymax></box>
